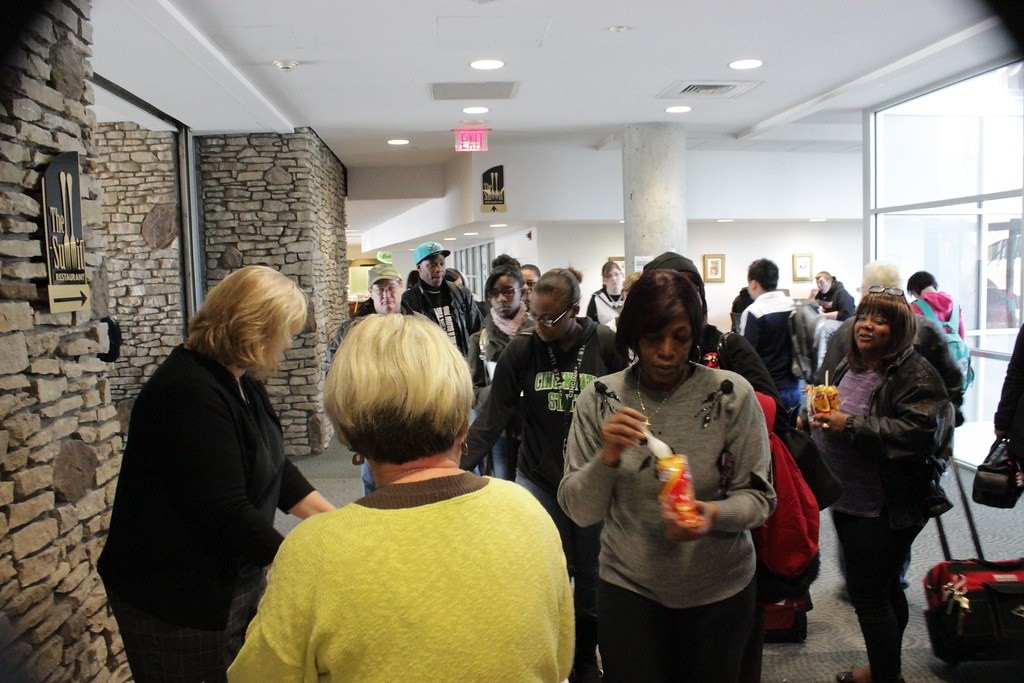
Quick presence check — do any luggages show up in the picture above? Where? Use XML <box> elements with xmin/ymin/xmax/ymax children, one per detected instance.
<box><xmin>924</xmin><ymin>455</ymin><xmax>1024</xmax><ymax>665</ymax></box>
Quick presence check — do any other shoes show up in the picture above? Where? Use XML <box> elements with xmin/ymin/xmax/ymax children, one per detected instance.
<box><xmin>836</xmin><ymin>666</ymin><xmax>906</xmax><ymax>683</ymax></box>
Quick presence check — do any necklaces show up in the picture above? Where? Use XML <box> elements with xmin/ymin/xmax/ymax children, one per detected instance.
<box><xmin>607</xmin><ymin>291</ymin><xmax>622</xmax><ymax>306</ymax></box>
<box><xmin>426</xmin><ymin>290</ymin><xmax>440</xmax><ymax>295</ymax></box>
<box><xmin>636</xmin><ymin>364</ymin><xmax>688</xmax><ymax>429</ymax></box>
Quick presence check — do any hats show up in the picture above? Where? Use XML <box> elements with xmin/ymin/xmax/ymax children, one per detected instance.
<box><xmin>413</xmin><ymin>242</ymin><xmax>451</xmax><ymax>265</ymax></box>
<box><xmin>368</xmin><ymin>263</ymin><xmax>403</xmax><ymax>287</ymax></box>
<box><xmin>643</xmin><ymin>251</ymin><xmax>701</xmax><ymax>276</ymax></box>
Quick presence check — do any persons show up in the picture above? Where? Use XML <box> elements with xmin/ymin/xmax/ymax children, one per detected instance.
<box><xmin>225</xmin><ymin>314</ymin><xmax>575</xmax><ymax>683</ymax></box>
<box><xmin>94</xmin><ymin>264</ymin><xmax>342</xmax><ymax>683</ymax></box>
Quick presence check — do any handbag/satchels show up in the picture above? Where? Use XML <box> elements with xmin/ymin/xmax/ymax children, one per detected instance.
<box><xmin>783</xmin><ymin>426</ymin><xmax>846</xmax><ymax>511</ymax></box>
<box><xmin>972</xmin><ymin>435</ymin><xmax>1024</xmax><ymax>509</ymax></box>
<box><xmin>764</xmin><ymin>594</ymin><xmax>813</xmax><ymax>644</ymax></box>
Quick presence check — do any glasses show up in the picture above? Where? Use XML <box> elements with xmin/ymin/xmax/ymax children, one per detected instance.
<box><xmin>606</xmin><ymin>273</ymin><xmax>623</xmax><ymax>277</ymax></box>
<box><xmin>523</xmin><ymin>281</ymin><xmax>535</xmax><ymax>287</ymax></box>
<box><xmin>488</xmin><ymin>288</ymin><xmax>522</xmax><ymax>298</ymax></box>
<box><xmin>869</xmin><ymin>285</ymin><xmax>907</xmax><ymax>303</ymax></box>
<box><xmin>525</xmin><ymin>301</ymin><xmax>580</xmax><ymax>328</ymax></box>
<box><xmin>868</xmin><ymin>286</ymin><xmax>911</xmax><ymax>308</ymax></box>
<box><xmin>856</xmin><ymin>287</ymin><xmax>866</xmax><ymax>293</ymax></box>
<box><xmin>370</xmin><ymin>286</ymin><xmax>402</xmax><ymax>294</ymax></box>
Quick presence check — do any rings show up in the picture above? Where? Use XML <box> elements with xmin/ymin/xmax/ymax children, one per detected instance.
<box><xmin>820</xmin><ymin>423</ymin><xmax>829</xmax><ymax>432</ymax></box>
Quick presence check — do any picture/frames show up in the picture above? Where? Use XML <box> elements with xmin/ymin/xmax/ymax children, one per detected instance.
<box><xmin>609</xmin><ymin>257</ymin><xmax>625</xmax><ymax>273</ymax></box>
<box><xmin>792</xmin><ymin>254</ymin><xmax>813</xmax><ymax>281</ymax></box>
<box><xmin>702</xmin><ymin>253</ymin><xmax>725</xmax><ymax>282</ymax></box>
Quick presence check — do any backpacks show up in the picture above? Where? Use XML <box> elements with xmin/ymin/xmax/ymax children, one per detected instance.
<box><xmin>789</xmin><ymin>300</ymin><xmax>827</xmax><ymax>382</ymax></box>
<box><xmin>910</xmin><ymin>298</ymin><xmax>974</xmax><ymax>396</ymax></box>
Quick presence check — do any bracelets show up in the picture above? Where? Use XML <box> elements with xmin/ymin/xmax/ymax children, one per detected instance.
<box><xmin>845</xmin><ymin>415</ymin><xmax>857</xmax><ymax>440</ymax></box>
<box><xmin>330</xmin><ymin>242</ymin><xmax>1024</xmax><ymax>683</ymax></box>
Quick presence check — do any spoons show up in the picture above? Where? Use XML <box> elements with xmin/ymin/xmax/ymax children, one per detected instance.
<box><xmin>641</xmin><ymin>428</ymin><xmax>673</xmax><ymax>459</ymax></box>
